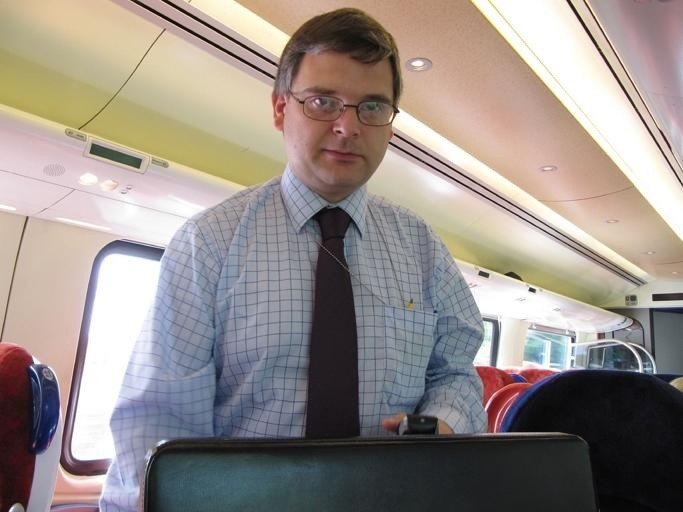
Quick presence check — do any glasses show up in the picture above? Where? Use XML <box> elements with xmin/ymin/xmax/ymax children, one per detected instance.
<box><xmin>286</xmin><ymin>90</ymin><xmax>399</xmax><ymax>127</ymax></box>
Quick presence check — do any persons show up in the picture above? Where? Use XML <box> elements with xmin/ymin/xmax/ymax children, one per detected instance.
<box><xmin>95</xmin><ymin>7</ymin><xmax>486</xmax><ymax>512</ymax></box>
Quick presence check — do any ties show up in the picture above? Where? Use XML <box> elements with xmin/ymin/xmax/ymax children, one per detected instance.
<box><xmin>304</xmin><ymin>207</ymin><xmax>361</xmax><ymax>437</ymax></box>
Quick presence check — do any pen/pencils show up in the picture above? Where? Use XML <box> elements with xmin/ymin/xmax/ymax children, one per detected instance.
<box><xmin>406</xmin><ymin>299</ymin><xmax>415</xmax><ymax>310</ymax></box>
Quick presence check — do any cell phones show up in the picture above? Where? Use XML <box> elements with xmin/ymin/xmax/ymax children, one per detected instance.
<box><xmin>397</xmin><ymin>415</ymin><xmax>440</xmax><ymax>437</ymax></box>
